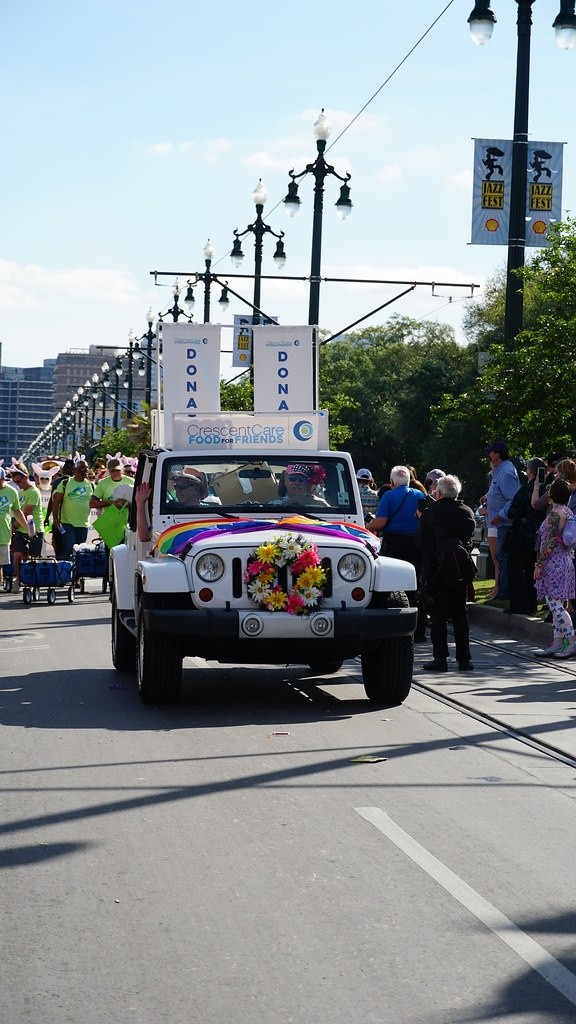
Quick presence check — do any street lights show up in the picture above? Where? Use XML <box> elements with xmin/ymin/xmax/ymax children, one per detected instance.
<box><xmin>467</xmin><ymin>0</ymin><xmax>576</xmax><ymax>353</ymax></box>
<box><xmin>282</xmin><ymin>108</ymin><xmax>353</xmax><ymax>326</ymax></box>
<box><xmin>229</xmin><ymin>179</ymin><xmax>288</xmax><ymax>325</ymax></box>
<box><xmin>20</xmin><ymin>236</ymin><xmax>230</xmax><ymax>464</ymax></box>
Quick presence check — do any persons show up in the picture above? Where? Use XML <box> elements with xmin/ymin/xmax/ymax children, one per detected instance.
<box><xmin>357</xmin><ymin>468</ymin><xmax>382</xmax><ymax>536</ymax></box>
<box><xmin>416</xmin><ymin>469</ymin><xmax>478</xmax><ymax>672</ymax></box>
<box><xmin>135</xmin><ymin>467</ymin><xmax>209</xmax><ymax>543</ymax></box>
<box><xmin>507</xmin><ymin>451</ymin><xmax>576</xmax><ymax>659</ymax></box>
<box><xmin>0</xmin><ymin>451</ymin><xmax>138</xmax><ymax>593</ymax></box>
<box><xmin>480</xmin><ymin>441</ymin><xmax>521</xmax><ymax>601</ymax></box>
<box><xmin>268</xmin><ymin>465</ymin><xmax>331</xmax><ymax>507</ymax></box>
<box><xmin>365</xmin><ymin>465</ymin><xmax>428</xmax><ymax>643</ymax></box>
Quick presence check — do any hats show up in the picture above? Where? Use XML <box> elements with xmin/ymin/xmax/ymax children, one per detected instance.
<box><xmin>484</xmin><ymin>442</ymin><xmax>507</xmax><ymax>456</ymax></box>
<box><xmin>284</xmin><ymin>464</ymin><xmax>312</xmax><ymax>479</ymax></box>
<box><xmin>172</xmin><ymin>465</ymin><xmax>202</xmax><ymax>485</ymax></box>
<box><xmin>357</xmin><ymin>469</ymin><xmax>372</xmax><ymax>481</ymax></box>
<box><xmin>107</xmin><ymin>458</ymin><xmax>122</xmax><ymax>471</ymax></box>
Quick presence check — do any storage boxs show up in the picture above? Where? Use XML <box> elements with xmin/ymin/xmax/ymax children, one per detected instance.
<box><xmin>73</xmin><ymin>550</ymin><xmax>109</xmax><ymax>577</ymax></box>
<box><xmin>3</xmin><ymin>552</ymin><xmax>17</xmax><ymax>577</ymax></box>
<box><xmin>210</xmin><ymin>461</ymin><xmax>279</xmax><ymax>504</ymax></box>
<box><xmin>20</xmin><ymin>562</ymin><xmax>72</xmax><ymax>585</ymax></box>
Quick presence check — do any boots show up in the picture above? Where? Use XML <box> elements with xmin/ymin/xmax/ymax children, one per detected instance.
<box><xmin>533</xmin><ymin>637</ymin><xmax>564</xmax><ymax>657</ymax></box>
<box><xmin>554</xmin><ymin>636</ymin><xmax>576</xmax><ymax>658</ymax></box>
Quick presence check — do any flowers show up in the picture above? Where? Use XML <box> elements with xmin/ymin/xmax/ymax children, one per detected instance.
<box><xmin>242</xmin><ymin>533</ymin><xmax>332</xmax><ymax>620</ymax></box>
<box><xmin>309</xmin><ymin>465</ymin><xmax>326</xmax><ymax>485</ymax></box>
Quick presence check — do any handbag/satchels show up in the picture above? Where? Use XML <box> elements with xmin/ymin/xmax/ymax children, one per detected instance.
<box><xmin>93</xmin><ymin>502</ymin><xmax>130</xmax><ymax>550</ymax></box>
<box><xmin>551</xmin><ymin>507</ymin><xmax>576</xmax><ymax>545</ymax></box>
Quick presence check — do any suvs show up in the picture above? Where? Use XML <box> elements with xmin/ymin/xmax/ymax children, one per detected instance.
<box><xmin>108</xmin><ymin>322</ymin><xmax>418</xmax><ymax>704</ymax></box>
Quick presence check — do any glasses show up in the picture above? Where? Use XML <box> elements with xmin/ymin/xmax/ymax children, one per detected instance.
<box><xmin>40</xmin><ymin>477</ymin><xmax>50</xmax><ymax>482</ymax></box>
<box><xmin>13</xmin><ymin>477</ymin><xmax>24</xmax><ymax>485</ymax></box>
<box><xmin>287</xmin><ymin>474</ymin><xmax>308</xmax><ymax>483</ymax></box>
<box><xmin>125</xmin><ymin>470</ymin><xmax>135</xmax><ymax>475</ymax></box>
<box><xmin>173</xmin><ymin>483</ymin><xmax>195</xmax><ymax>491</ymax></box>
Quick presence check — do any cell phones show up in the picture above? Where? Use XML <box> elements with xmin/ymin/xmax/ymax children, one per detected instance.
<box><xmin>538</xmin><ymin>467</ymin><xmax>545</xmax><ymax>484</ymax></box>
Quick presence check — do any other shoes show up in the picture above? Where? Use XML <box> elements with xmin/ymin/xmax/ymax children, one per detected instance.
<box><xmin>460</xmin><ymin>662</ymin><xmax>475</xmax><ymax>671</ymax></box>
<box><xmin>415</xmin><ymin>635</ymin><xmax>426</xmax><ymax>643</ymax></box>
<box><xmin>423</xmin><ymin>658</ymin><xmax>448</xmax><ymax>671</ymax></box>
<box><xmin>482</xmin><ymin>590</ymin><xmax>534</xmax><ymax>616</ymax></box>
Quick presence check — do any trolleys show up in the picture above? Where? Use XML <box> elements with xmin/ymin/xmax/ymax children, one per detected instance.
<box><xmin>3</xmin><ymin>532</ymin><xmax>108</xmax><ymax>604</ymax></box>
